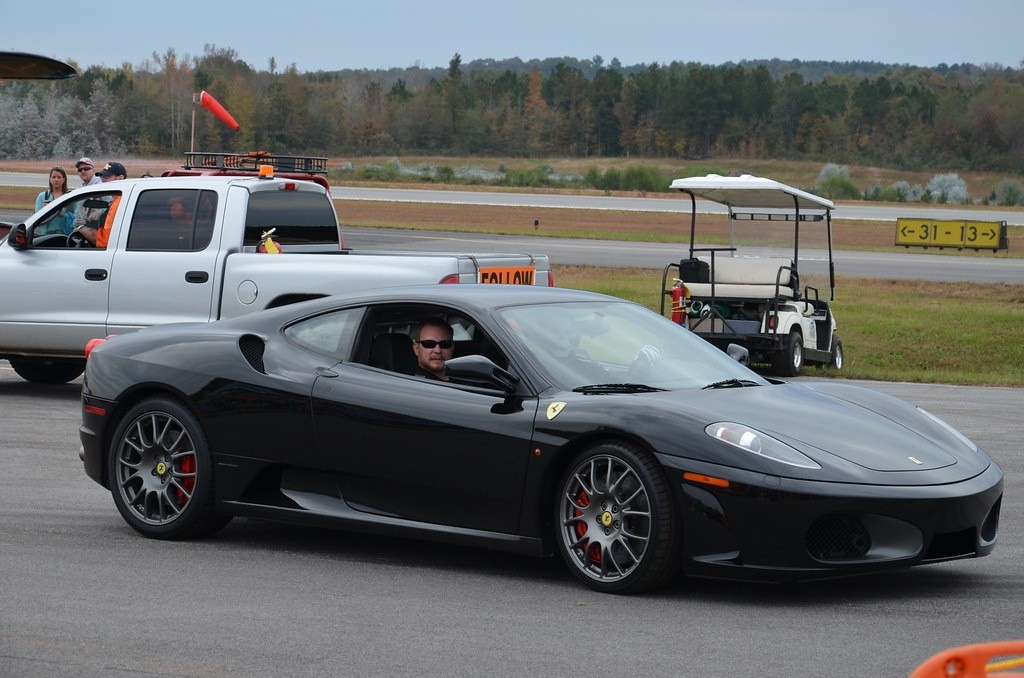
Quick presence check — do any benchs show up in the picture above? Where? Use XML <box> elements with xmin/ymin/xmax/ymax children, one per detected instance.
<box><xmin>681</xmin><ymin>256</ymin><xmax>794</xmax><ymax>300</ymax></box>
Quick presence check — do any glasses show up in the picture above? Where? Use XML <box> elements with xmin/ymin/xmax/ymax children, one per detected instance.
<box><xmin>416</xmin><ymin>339</ymin><xmax>453</xmax><ymax>349</ymax></box>
<box><xmin>78</xmin><ymin>167</ymin><xmax>92</xmax><ymax>172</ymax></box>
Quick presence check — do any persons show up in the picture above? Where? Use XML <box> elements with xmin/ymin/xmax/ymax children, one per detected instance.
<box><xmin>545</xmin><ymin>318</ymin><xmax>662</xmax><ymax>384</ymax></box>
<box><xmin>35</xmin><ymin>166</ymin><xmax>75</xmax><ymax>235</ymax></box>
<box><xmin>73</xmin><ymin>224</ymin><xmax>111</xmax><ymax>248</ymax></box>
<box><xmin>75</xmin><ymin>156</ymin><xmax>112</xmax><ymax>223</ymax></box>
<box><xmin>95</xmin><ymin>162</ymin><xmax>127</xmax><ymax>230</ymax></box>
<box><xmin>406</xmin><ymin>317</ymin><xmax>482</xmax><ymax>387</ymax></box>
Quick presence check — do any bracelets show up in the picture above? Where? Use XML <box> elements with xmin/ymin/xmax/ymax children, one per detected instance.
<box><xmin>78</xmin><ymin>225</ymin><xmax>84</xmax><ymax>230</ymax></box>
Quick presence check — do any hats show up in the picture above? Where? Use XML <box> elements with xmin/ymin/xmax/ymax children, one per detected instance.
<box><xmin>75</xmin><ymin>158</ymin><xmax>95</xmax><ymax>168</ymax></box>
<box><xmin>95</xmin><ymin>162</ymin><xmax>127</xmax><ymax>179</ymax></box>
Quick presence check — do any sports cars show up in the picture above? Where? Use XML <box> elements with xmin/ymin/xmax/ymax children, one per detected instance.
<box><xmin>77</xmin><ymin>282</ymin><xmax>1003</xmax><ymax>598</ymax></box>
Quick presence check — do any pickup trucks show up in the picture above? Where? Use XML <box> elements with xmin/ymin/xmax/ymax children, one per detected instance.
<box><xmin>0</xmin><ymin>176</ymin><xmax>555</xmax><ymax>385</ymax></box>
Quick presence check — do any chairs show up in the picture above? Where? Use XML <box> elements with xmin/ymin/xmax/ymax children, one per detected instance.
<box><xmin>370</xmin><ymin>333</ymin><xmax>420</xmax><ymax>378</ymax></box>
